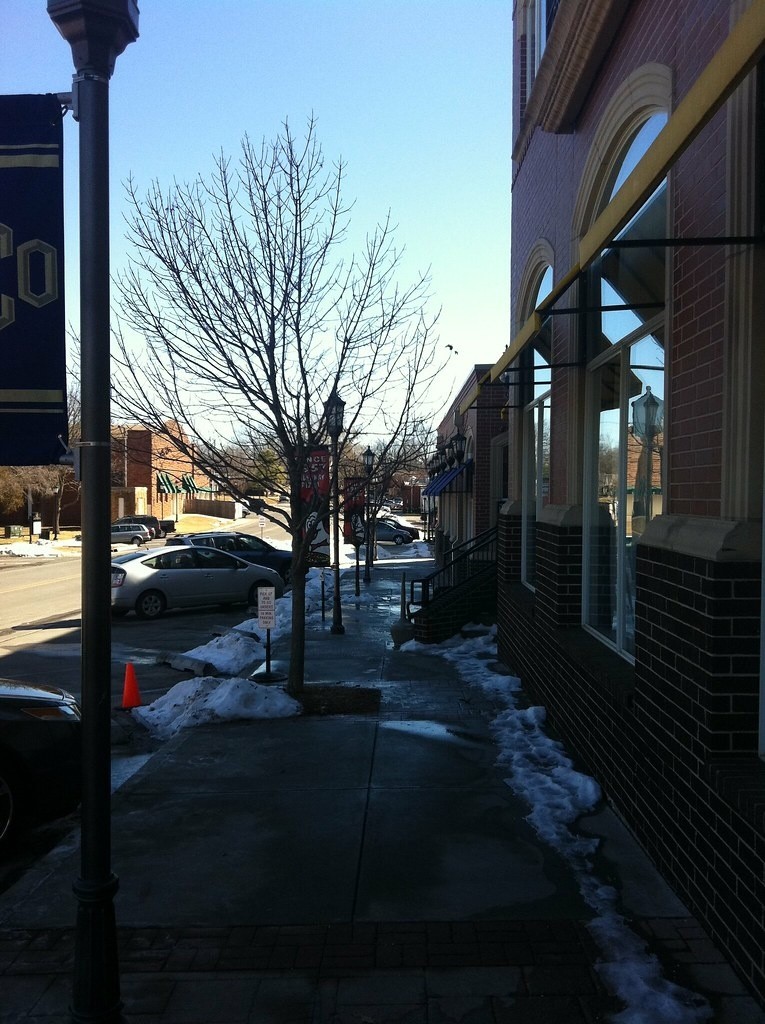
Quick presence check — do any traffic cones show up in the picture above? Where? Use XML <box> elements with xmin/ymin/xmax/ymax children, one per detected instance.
<box><xmin>112</xmin><ymin>662</ymin><xmax>151</xmax><ymax>711</ymax></box>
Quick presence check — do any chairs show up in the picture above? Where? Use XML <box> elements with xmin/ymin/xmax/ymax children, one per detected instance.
<box><xmin>227</xmin><ymin>539</ymin><xmax>236</xmax><ymax>550</ymax></box>
<box><xmin>180</xmin><ymin>555</ymin><xmax>193</xmax><ymax>569</ymax></box>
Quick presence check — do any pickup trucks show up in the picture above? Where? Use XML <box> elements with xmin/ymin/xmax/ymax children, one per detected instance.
<box><xmin>156</xmin><ymin>520</ymin><xmax>175</xmax><ymax>538</ymax></box>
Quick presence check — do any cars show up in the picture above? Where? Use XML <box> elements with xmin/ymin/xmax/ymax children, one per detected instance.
<box><xmin>0</xmin><ymin>679</ymin><xmax>87</xmax><ymax>847</ymax></box>
<box><xmin>110</xmin><ymin>544</ymin><xmax>284</xmax><ymax>620</ymax></box>
<box><xmin>368</xmin><ymin>498</ymin><xmax>422</xmax><ymax>545</ymax></box>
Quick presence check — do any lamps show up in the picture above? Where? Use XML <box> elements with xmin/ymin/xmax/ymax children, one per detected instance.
<box><xmin>426</xmin><ymin>428</ymin><xmax>468</xmax><ymax>479</ymax></box>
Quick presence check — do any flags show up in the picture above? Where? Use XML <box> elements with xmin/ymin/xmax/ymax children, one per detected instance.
<box><xmin>0</xmin><ymin>93</ymin><xmax>69</xmax><ymax>467</ymax></box>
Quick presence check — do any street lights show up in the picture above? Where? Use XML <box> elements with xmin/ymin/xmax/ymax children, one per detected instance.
<box><xmin>363</xmin><ymin>446</ymin><xmax>377</xmax><ymax>579</ymax></box>
<box><xmin>629</xmin><ymin>387</ymin><xmax>665</xmax><ymax>527</ymax></box>
<box><xmin>44</xmin><ymin>1</ymin><xmax>141</xmax><ymax>1022</ymax></box>
<box><xmin>322</xmin><ymin>391</ymin><xmax>347</xmax><ymax>633</ymax></box>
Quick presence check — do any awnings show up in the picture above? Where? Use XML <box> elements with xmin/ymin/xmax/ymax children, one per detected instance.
<box><xmin>533</xmin><ymin>317</ymin><xmax>642</xmax><ymax>414</ymax></box>
<box><xmin>599</xmin><ymin>192</ymin><xmax>668</xmax><ymax>351</ymax></box>
<box><xmin>157</xmin><ymin>473</ymin><xmax>198</xmax><ymax>493</ymax></box>
<box><xmin>580</xmin><ymin>0</ymin><xmax>765</xmax><ymax>270</ymax></box>
<box><xmin>490</xmin><ymin>262</ymin><xmax>667</xmax><ymax>381</ymax></box>
<box><xmin>422</xmin><ymin>458</ymin><xmax>473</xmax><ymax>496</ymax></box>
<box><xmin>461</xmin><ymin>372</ymin><xmax>521</xmax><ymax>417</ymax></box>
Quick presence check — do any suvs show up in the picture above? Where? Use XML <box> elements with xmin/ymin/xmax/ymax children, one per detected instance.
<box><xmin>240</xmin><ymin>500</ymin><xmax>251</xmax><ymax>518</ymax></box>
<box><xmin>112</xmin><ymin>514</ymin><xmax>163</xmax><ymax>539</ymax></box>
<box><xmin>111</xmin><ymin>523</ymin><xmax>151</xmax><ymax>545</ymax></box>
<box><xmin>167</xmin><ymin>531</ymin><xmax>311</xmax><ymax>581</ymax></box>
<box><xmin>251</xmin><ymin>499</ymin><xmax>267</xmax><ymax>512</ymax></box>
<box><xmin>279</xmin><ymin>495</ymin><xmax>289</xmax><ymax>502</ymax></box>
